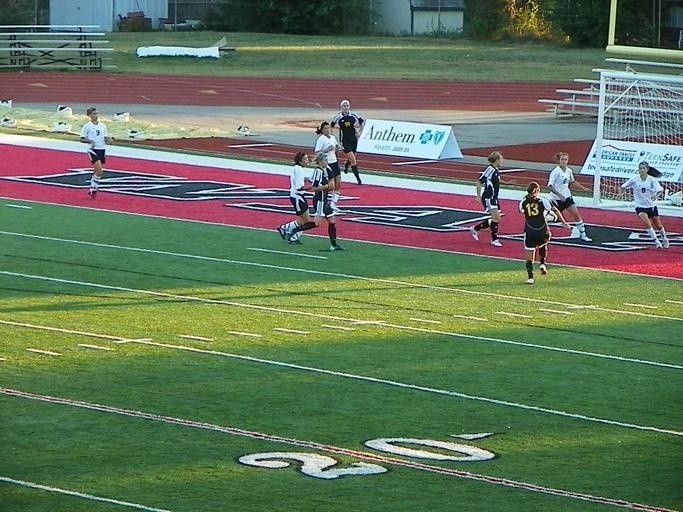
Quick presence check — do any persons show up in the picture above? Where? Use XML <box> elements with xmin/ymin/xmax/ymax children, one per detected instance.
<box><xmin>286</xmin><ymin>152</ymin><xmax>343</xmax><ymax>251</ymax></box>
<box><xmin>327</xmin><ymin>100</ymin><xmax>366</xmax><ymax>185</ymax></box>
<box><xmin>469</xmin><ymin>152</ymin><xmax>516</xmax><ymax>247</ymax></box>
<box><xmin>518</xmin><ymin>181</ymin><xmax>550</xmax><ymax>284</ymax></box>
<box><xmin>615</xmin><ymin>162</ymin><xmax>668</xmax><ymax>250</ymax></box>
<box><xmin>278</xmin><ymin>152</ymin><xmax>313</xmax><ymax>244</ymax></box>
<box><xmin>77</xmin><ymin>106</ymin><xmax>107</xmax><ymax>200</ymax></box>
<box><xmin>312</xmin><ymin>122</ymin><xmax>344</xmax><ymax>211</ymax></box>
<box><xmin>547</xmin><ymin>152</ymin><xmax>593</xmax><ymax>242</ymax></box>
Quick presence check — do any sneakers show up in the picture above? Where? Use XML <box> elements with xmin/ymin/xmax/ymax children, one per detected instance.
<box><xmin>470</xmin><ymin>226</ymin><xmax>479</xmax><ymax>241</ymax></box>
<box><xmin>490</xmin><ymin>239</ymin><xmax>503</xmax><ymax>247</ymax></box>
<box><xmin>275</xmin><ymin>224</ymin><xmax>303</xmax><ymax>246</ymax></box>
<box><xmin>655</xmin><ymin>243</ymin><xmax>663</xmax><ymax>250</ymax></box>
<box><xmin>539</xmin><ymin>264</ymin><xmax>548</xmax><ymax>275</ymax></box>
<box><xmin>330</xmin><ymin>244</ymin><xmax>346</xmax><ymax>252</ymax></box>
<box><xmin>526</xmin><ymin>278</ymin><xmax>535</xmax><ymax>284</ymax></box>
<box><xmin>88</xmin><ymin>189</ymin><xmax>99</xmax><ymax>200</ymax></box>
<box><xmin>581</xmin><ymin>236</ymin><xmax>593</xmax><ymax>242</ymax></box>
<box><xmin>663</xmin><ymin>239</ymin><xmax>670</xmax><ymax>249</ymax></box>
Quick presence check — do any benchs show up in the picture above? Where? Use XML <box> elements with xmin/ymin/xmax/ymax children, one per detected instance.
<box><xmin>537</xmin><ymin>56</ymin><xmax>683</xmax><ymax>129</ymax></box>
<box><xmin>0</xmin><ymin>25</ymin><xmax>117</xmax><ymax>72</ymax></box>
